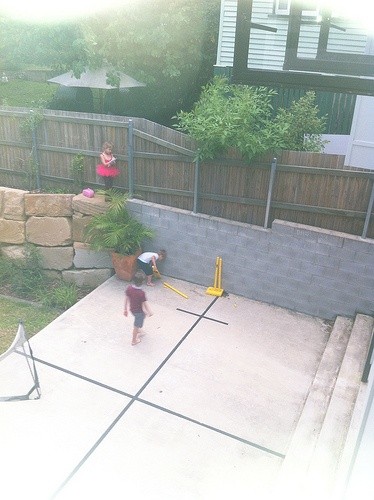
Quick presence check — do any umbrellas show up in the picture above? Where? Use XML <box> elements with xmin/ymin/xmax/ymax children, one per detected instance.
<box><xmin>47</xmin><ymin>64</ymin><xmax>150</xmax><ymax>91</ymax></box>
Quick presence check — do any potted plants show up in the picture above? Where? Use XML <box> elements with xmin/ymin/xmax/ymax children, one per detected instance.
<box><xmin>82</xmin><ymin>184</ymin><xmax>158</xmax><ymax>281</ymax></box>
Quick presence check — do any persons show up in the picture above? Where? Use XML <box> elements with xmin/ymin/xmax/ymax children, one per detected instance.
<box><xmin>122</xmin><ymin>271</ymin><xmax>154</xmax><ymax>346</ymax></box>
<box><xmin>95</xmin><ymin>139</ymin><xmax>119</xmax><ymax>192</ymax></box>
<box><xmin>136</xmin><ymin>248</ymin><xmax>165</xmax><ymax>286</ymax></box>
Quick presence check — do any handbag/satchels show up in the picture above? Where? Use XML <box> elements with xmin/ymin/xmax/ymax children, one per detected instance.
<box><xmin>83</xmin><ymin>188</ymin><xmax>94</xmax><ymax>197</ymax></box>
